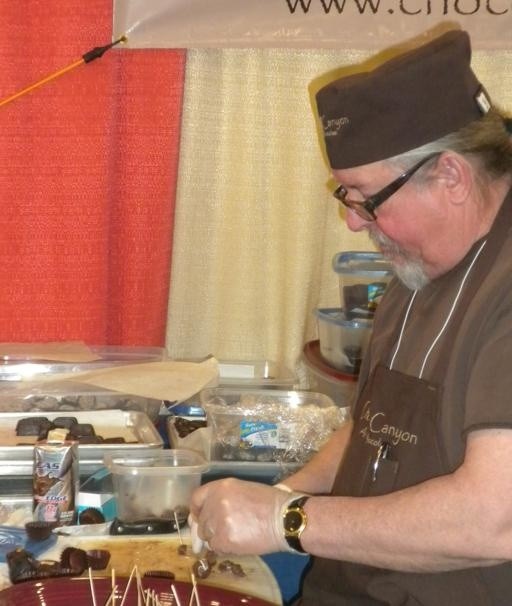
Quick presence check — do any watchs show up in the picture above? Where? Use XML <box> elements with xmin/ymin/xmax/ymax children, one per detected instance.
<box><xmin>281</xmin><ymin>496</ymin><xmax>309</xmax><ymax>555</ymax></box>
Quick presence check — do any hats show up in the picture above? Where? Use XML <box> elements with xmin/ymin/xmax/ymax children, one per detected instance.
<box><xmin>315</xmin><ymin>30</ymin><xmax>491</xmax><ymax>169</ymax></box>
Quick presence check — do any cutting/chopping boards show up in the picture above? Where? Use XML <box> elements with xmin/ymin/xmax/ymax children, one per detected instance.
<box><xmin>1</xmin><ymin>534</ymin><xmax>282</xmax><ymax>606</ymax></box>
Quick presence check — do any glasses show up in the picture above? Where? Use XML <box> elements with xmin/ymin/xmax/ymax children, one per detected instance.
<box><xmin>332</xmin><ymin>151</ymin><xmax>440</xmax><ymax>221</ymax></box>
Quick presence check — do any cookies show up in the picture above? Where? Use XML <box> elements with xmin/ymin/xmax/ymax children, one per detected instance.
<box><xmin>0</xmin><ymin>392</ymin><xmax>246</xmax><ymax>586</ymax></box>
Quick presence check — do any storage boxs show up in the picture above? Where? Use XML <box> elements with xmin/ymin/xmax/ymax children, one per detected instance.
<box><xmin>300</xmin><ymin>251</ymin><xmax>394</xmax><ymax>405</ymax></box>
<box><xmin>0</xmin><ymin>346</ymin><xmax>340</xmax><ymax>523</ymax></box>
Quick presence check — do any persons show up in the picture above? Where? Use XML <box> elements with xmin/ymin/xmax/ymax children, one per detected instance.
<box><xmin>190</xmin><ymin>31</ymin><xmax>512</xmax><ymax>606</ymax></box>
